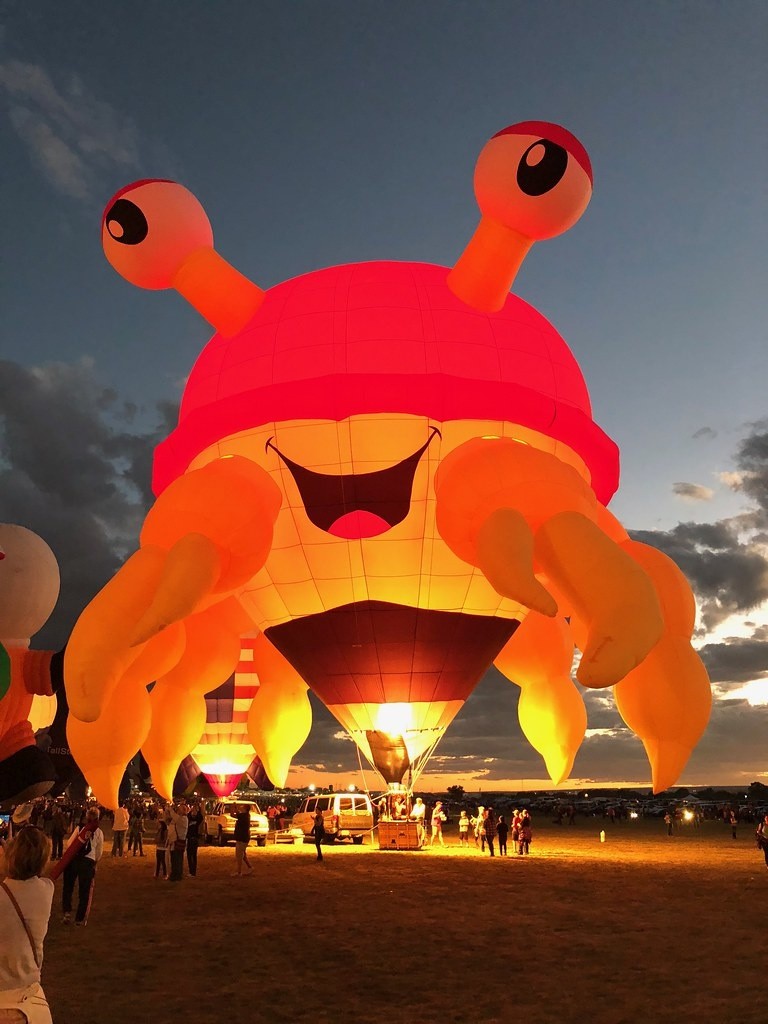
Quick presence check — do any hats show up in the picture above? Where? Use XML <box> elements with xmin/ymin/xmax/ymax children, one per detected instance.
<box><xmin>512</xmin><ymin>809</ymin><xmax>519</xmax><ymax>815</ymax></box>
<box><xmin>0</xmin><ymin>818</ymin><xmax>3</xmax><ymax>825</ymax></box>
<box><xmin>13</xmin><ymin>803</ymin><xmax>33</xmax><ymax>823</ymax></box>
<box><xmin>435</xmin><ymin>801</ymin><xmax>442</xmax><ymax>805</ymax></box>
<box><xmin>477</xmin><ymin>805</ymin><xmax>484</xmax><ymax>810</ymax></box>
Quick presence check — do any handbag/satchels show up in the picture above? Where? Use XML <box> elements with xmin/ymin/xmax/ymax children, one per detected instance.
<box><xmin>316</xmin><ymin>826</ymin><xmax>327</xmax><ymax>838</ymax></box>
<box><xmin>174</xmin><ymin>822</ymin><xmax>186</xmax><ymax>852</ymax></box>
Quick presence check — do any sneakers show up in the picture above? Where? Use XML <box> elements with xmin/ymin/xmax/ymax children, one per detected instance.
<box><xmin>51</xmin><ymin>857</ymin><xmax>55</xmax><ymax>861</ymax></box>
<box><xmin>245</xmin><ymin>867</ymin><xmax>254</xmax><ymax>874</ymax></box>
<box><xmin>73</xmin><ymin>921</ymin><xmax>82</xmax><ymax>928</ymax></box>
<box><xmin>62</xmin><ymin>913</ymin><xmax>71</xmax><ymax>924</ymax></box>
<box><xmin>153</xmin><ymin>872</ymin><xmax>158</xmax><ymax>880</ymax></box>
<box><xmin>186</xmin><ymin>874</ymin><xmax>195</xmax><ymax>878</ymax></box>
<box><xmin>230</xmin><ymin>872</ymin><xmax>242</xmax><ymax>876</ymax></box>
<box><xmin>164</xmin><ymin>874</ymin><xmax>168</xmax><ymax>880</ymax></box>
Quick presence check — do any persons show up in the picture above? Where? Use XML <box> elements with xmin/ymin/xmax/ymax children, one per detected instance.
<box><xmin>0</xmin><ymin>825</ymin><xmax>54</xmax><ymax>1024</ymax></box>
<box><xmin>0</xmin><ymin>792</ymin><xmax>768</xmax><ymax>927</ymax></box>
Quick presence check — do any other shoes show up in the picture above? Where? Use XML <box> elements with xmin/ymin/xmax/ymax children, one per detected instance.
<box><xmin>504</xmin><ymin>854</ymin><xmax>506</xmax><ymax>856</ymax></box>
<box><xmin>524</xmin><ymin>852</ymin><xmax>529</xmax><ymax>854</ymax></box>
<box><xmin>518</xmin><ymin>853</ymin><xmax>523</xmax><ymax>855</ymax></box>
<box><xmin>169</xmin><ymin>874</ymin><xmax>176</xmax><ymax>881</ymax></box>
<box><xmin>119</xmin><ymin>855</ymin><xmax>127</xmax><ymax>858</ymax></box>
<box><xmin>176</xmin><ymin>874</ymin><xmax>182</xmax><ymax>881</ymax></box>
<box><xmin>56</xmin><ymin>857</ymin><xmax>62</xmax><ymax>859</ymax></box>
<box><xmin>140</xmin><ymin>853</ymin><xmax>147</xmax><ymax>856</ymax></box>
<box><xmin>499</xmin><ymin>854</ymin><xmax>502</xmax><ymax>856</ymax></box>
<box><xmin>317</xmin><ymin>856</ymin><xmax>322</xmax><ymax>860</ymax></box>
<box><xmin>111</xmin><ymin>854</ymin><xmax>116</xmax><ymax>857</ymax></box>
<box><xmin>491</xmin><ymin>854</ymin><xmax>494</xmax><ymax>856</ymax></box>
<box><xmin>133</xmin><ymin>854</ymin><xmax>137</xmax><ymax>856</ymax></box>
<box><xmin>513</xmin><ymin>851</ymin><xmax>517</xmax><ymax>853</ymax></box>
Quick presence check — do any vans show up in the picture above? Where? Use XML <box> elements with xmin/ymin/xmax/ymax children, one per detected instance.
<box><xmin>291</xmin><ymin>793</ymin><xmax>374</xmax><ymax>844</ymax></box>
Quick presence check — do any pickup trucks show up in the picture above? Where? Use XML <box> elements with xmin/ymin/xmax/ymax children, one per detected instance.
<box><xmin>202</xmin><ymin>800</ymin><xmax>270</xmax><ymax>847</ymax></box>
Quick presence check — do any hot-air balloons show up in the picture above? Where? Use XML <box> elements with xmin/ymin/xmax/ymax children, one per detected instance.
<box><xmin>0</xmin><ymin>520</ymin><xmax>67</xmax><ymax>815</ymax></box>
<box><xmin>64</xmin><ymin>120</ymin><xmax>714</xmax><ymax>853</ymax></box>
